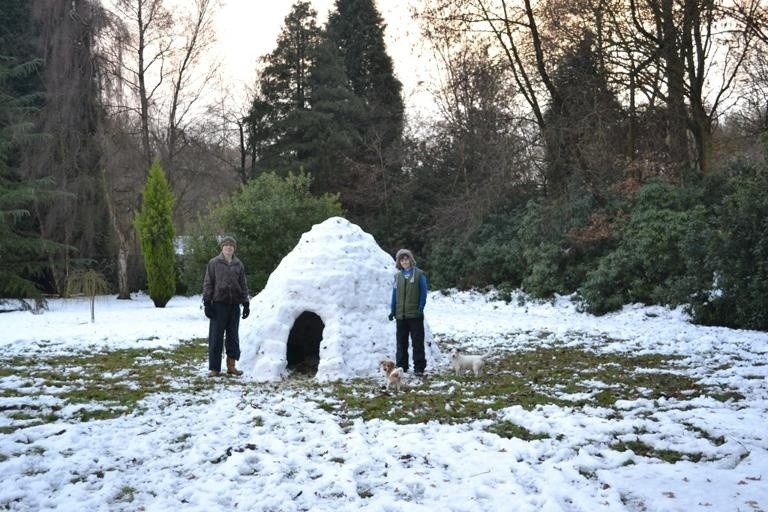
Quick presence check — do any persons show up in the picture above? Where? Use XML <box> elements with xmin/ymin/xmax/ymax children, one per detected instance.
<box><xmin>388</xmin><ymin>249</ymin><xmax>428</xmax><ymax>380</ymax></box>
<box><xmin>202</xmin><ymin>235</ymin><xmax>251</xmax><ymax>375</ymax></box>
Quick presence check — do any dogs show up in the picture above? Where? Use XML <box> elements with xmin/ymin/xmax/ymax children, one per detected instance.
<box><xmin>449</xmin><ymin>344</ymin><xmax>492</xmax><ymax>379</ymax></box>
<box><xmin>379</xmin><ymin>360</ymin><xmax>403</xmax><ymax>393</ymax></box>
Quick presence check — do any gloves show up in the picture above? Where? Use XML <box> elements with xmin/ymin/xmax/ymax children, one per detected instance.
<box><xmin>242</xmin><ymin>302</ymin><xmax>250</xmax><ymax>319</ymax></box>
<box><xmin>389</xmin><ymin>313</ymin><xmax>396</xmax><ymax>321</ymax></box>
<box><xmin>417</xmin><ymin>311</ymin><xmax>424</xmax><ymax>318</ymax></box>
<box><xmin>203</xmin><ymin>303</ymin><xmax>213</xmax><ymax>318</ymax></box>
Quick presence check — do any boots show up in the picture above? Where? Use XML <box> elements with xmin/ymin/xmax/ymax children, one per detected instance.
<box><xmin>227</xmin><ymin>358</ymin><xmax>243</xmax><ymax>376</ymax></box>
<box><xmin>207</xmin><ymin>370</ymin><xmax>219</xmax><ymax>377</ymax></box>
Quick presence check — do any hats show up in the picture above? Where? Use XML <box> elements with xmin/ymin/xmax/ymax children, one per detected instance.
<box><xmin>220</xmin><ymin>236</ymin><xmax>237</xmax><ymax>253</ymax></box>
<box><xmin>395</xmin><ymin>249</ymin><xmax>416</xmax><ymax>270</ymax></box>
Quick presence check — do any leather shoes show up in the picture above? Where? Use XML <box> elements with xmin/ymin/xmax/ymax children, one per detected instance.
<box><xmin>416</xmin><ymin>371</ymin><xmax>423</xmax><ymax>375</ymax></box>
